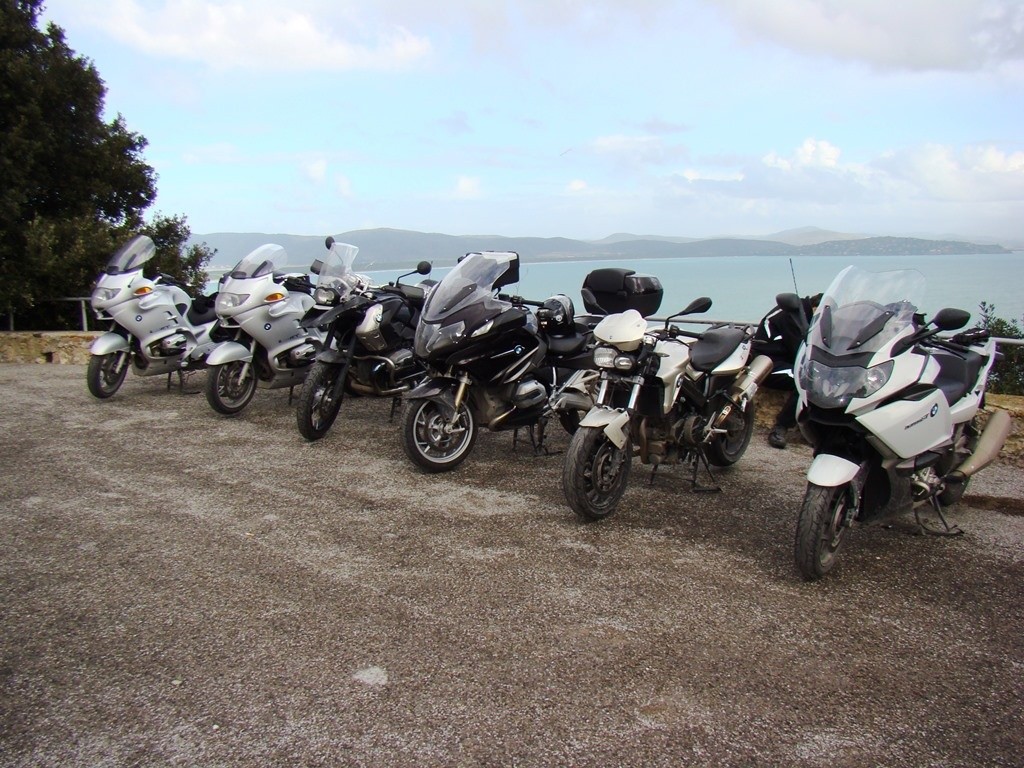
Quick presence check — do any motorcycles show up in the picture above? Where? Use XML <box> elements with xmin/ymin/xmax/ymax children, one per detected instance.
<box><xmin>296</xmin><ymin>236</ymin><xmax>443</xmax><ymax>442</ymax></box>
<box><xmin>775</xmin><ymin>261</ymin><xmax>1013</xmax><ymax>584</ymax></box>
<box><xmin>83</xmin><ymin>236</ymin><xmax>227</xmax><ymax>399</ymax></box>
<box><xmin>375</xmin><ymin>249</ymin><xmax>664</xmax><ymax>476</ymax></box>
<box><xmin>541</xmin><ymin>284</ymin><xmax>775</xmax><ymax>523</ymax></box>
<box><xmin>201</xmin><ymin>241</ymin><xmax>348</xmax><ymax>419</ymax></box>
